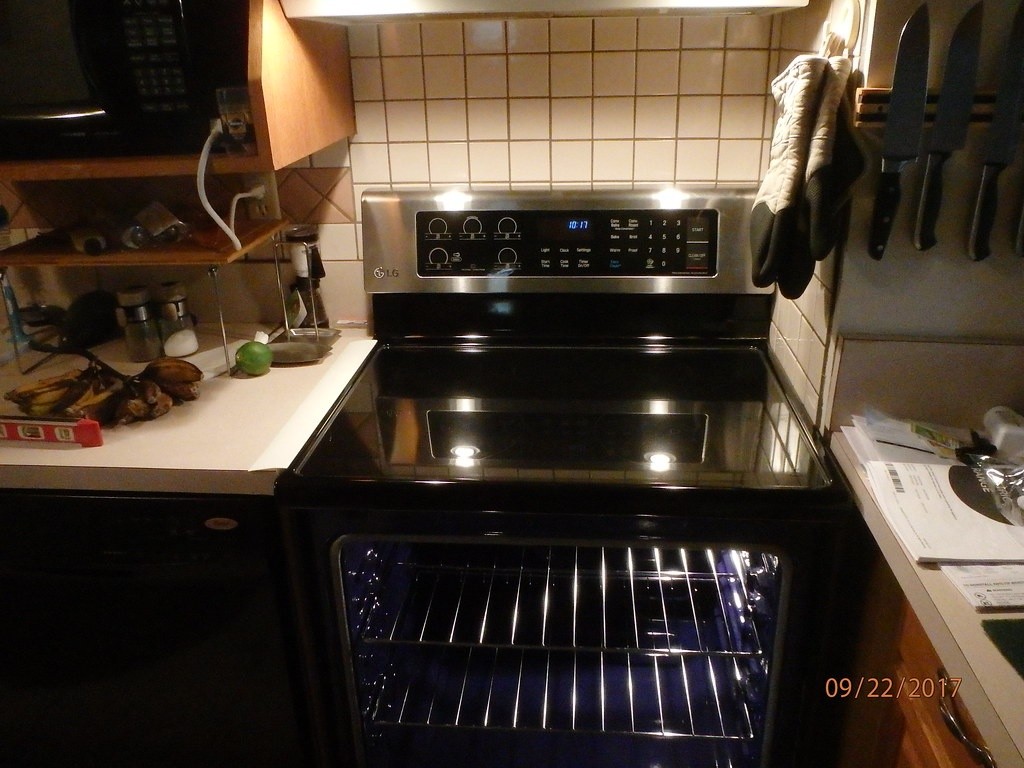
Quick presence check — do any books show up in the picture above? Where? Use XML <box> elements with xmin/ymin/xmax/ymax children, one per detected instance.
<box><xmin>840</xmin><ymin>417</ymin><xmax>1024</xmax><ymax>564</ymax></box>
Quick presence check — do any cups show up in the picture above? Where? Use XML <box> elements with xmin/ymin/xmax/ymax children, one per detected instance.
<box><xmin>212</xmin><ymin>86</ymin><xmax>257</xmax><ymax>155</ymax></box>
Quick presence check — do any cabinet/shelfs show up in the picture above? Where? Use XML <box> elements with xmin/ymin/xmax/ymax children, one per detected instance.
<box><xmin>839</xmin><ymin>543</ymin><xmax>996</xmax><ymax>767</ymax></box>
<box><xmin>0</xmin><ymin>0</ymin><xmax>357</xmax><ymax>268</ymax></box>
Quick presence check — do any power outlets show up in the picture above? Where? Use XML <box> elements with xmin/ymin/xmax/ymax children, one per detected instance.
<box><xmin>241</xmin><ymin>171</ymin><xmax>283</xmax><ymax>221</ymax></box>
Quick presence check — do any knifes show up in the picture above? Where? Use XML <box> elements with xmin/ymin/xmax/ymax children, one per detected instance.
<box><xmin>912</xmin><ymin>0</ymin><xmax>985</xmax><ymax>251</ymax></box>
<box><xmin>968</xmin><ymin>0</ymin><xmax>1024</xmax><ymax>262</ymax></box>
<box><xmin>867</xmin><ymin>0</ymin><xmax>931</xmax><ymax>261</ymax></box>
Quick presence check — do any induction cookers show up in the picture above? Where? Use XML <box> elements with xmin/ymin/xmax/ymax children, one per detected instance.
<box><xmin>291</xmin><ymin>187</ymin><xmax>837</xmax><ymax>489</ymax></box>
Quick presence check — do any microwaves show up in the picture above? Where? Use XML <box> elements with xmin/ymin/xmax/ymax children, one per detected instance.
<box><xmin>0</xmin><ymin>0</ymin><xmax>222</xmax><ymax>160</ymax></box>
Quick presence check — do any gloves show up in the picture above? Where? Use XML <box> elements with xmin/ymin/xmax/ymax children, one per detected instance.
<box><xmin>748</xmin><ymin>57</ymin><xmax>866</xmax><ymax>300</ymax></box>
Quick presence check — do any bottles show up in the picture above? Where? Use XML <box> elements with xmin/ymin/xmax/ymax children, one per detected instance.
<box><xmin>285</xmin><ymin>226</ymin><xmax>329</xmax><ymax>328</ymax></box>
<box><xmin>156</xmin><ymin>281</ymin><xmax>200</xmax><ymax>357</ymax></box>
<box><xmin>117</xmin><ymin>285</ymin><xmax>165</xmax><ymax>363</ymax></box>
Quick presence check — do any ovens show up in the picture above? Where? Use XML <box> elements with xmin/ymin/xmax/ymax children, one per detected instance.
<box><xmin>286</xmin><ymin>478</ymin><xmax>852</xmax><ymax>768</ymax></box>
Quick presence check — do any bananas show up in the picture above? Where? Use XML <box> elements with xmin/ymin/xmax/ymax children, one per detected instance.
<box><xmin>2</xmin><ymin>358</ymin><xmax>204</xmax><ymax>425</ymax></box>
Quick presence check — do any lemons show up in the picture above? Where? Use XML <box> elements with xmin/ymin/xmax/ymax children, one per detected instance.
<box><xmin>234</xmin><ymin>341</ymin><xmax>274</xmax><ymax>376</ymax></box>
